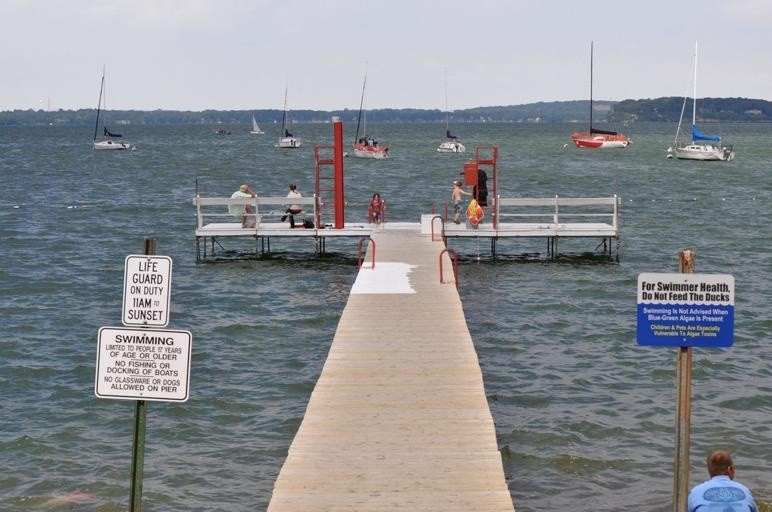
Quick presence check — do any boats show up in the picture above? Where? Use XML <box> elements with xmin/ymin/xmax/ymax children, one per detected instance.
<box><xmin>212</xmin><ymin>128</ymin><xmax>233</xmax><ymax>138</ymax></box>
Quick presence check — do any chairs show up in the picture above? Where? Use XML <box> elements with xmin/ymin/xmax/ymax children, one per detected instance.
<box><xmin>368</xmin><ymin>198</ymin><xmax>384</xmax><ymax>224</ymax></box>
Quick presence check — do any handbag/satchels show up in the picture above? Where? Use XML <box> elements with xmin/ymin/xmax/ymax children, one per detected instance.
<box><xmin>302</xmin><ymin>217</ymin><xmax>314</xmax><ymax>228</ymax></box>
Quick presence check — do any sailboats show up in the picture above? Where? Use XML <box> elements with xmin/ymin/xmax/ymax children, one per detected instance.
<box><xmin>664</xmin><ymin>40</ymin><xmax>744</xmax><ymax>162</ymax></box>
<box><xmin>349</xmin><ymin>63</ymin><xmax>391</xmax><ymax>159</ymax></box>
<box><xmin>272</xmin><ymin>88</ymin><xmax>303</xmax><ymax>148</ymax></box>
<box><xmin>249</xmin><ymin>113</ymin><xmax>267</xmax><ymax>136</ymax></box>
<box><xmin>437</xmin><ymin>66</ymin><xmax>466</xmax><ymax>153</ymax></box>
<box><xmin>90</xmin><ymin>60</ymin><xmax>139</xmax><ymax>154</ymax></box>
<box><xmin>570</xmin><ymin>39</ymin><xmax>633</xmax><ymax>151</ymax></box>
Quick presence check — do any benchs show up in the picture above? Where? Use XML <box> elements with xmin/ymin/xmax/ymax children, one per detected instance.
<box><xmin>492</xmin><ymin>194</ymin><xmax>621</xmax><ymax>231</ymax></box>
<box><xmin>192</xmin><ymin>194</ymin><xmax>323</xmax><ymax>231</ymax></box>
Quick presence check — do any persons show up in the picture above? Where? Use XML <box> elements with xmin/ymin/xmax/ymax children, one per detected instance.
<box><xmin>368</xmin><ymin>192</ymin><xmax>386</xmax><ymax>224</ymax></box>
<box><xmin>228</xmin><ymin>184</ymin><xmax>259</xmax><ymax>227</ymax></box>
<box><xmin>473</xmin><ymin>169</ymin><xmax>488</xmax><ymax>222</ymax></box>
<box><xmin>281</xmin><ymin>184</ymin><xmax>304</xmax><ymax>227</ymax></box>
<box><xmin>354</xmin><ymin>137</ymin><xmax>385</xmax><ymax>154</ymax></box>
<box><xmin>451</xmin><ymin>179</ymin><xmax>473</xmax><ymax>224</ymax></box>
<box><xmin>688</xmin><ymin>449</ymin><xmax>757</xmax><ymax>511</ymax></box>
